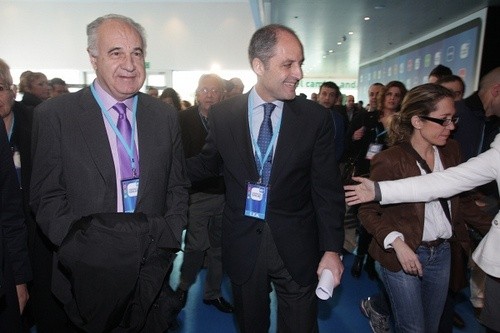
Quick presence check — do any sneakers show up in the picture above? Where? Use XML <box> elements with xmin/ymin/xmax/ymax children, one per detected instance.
<box><xmin>360</xmin><ymin>298</ymin><xmax>393</xmax><ymax>333</ymax></box>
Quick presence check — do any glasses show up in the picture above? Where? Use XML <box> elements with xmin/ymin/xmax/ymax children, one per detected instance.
<box><xmin>201</xmin><ymin>87</ymin><xmax>218</xmax><ymax>94</ymax></box>
<box><xmin>419</xmin><ymin>115</ymin><xmax>461</xmax><ymax>126</ymax></box>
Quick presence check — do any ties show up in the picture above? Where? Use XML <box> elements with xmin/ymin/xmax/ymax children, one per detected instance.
<box><xmin>253</xmin><ymin>103</ymin><xmax>276</xmax><ymax>186</ymax></box>
<box><xmin>112</xmin><ymin>103</ymin><xmax>139</xmax><ymax>181</ymax></box>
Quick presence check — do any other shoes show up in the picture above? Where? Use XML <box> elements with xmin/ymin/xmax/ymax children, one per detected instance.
<box><xmin>452</xmin><ymin>311</ymin><xmax>466</xmax><ymax>329</ymax></box>
<box><xmin>364</xmin><ymin>259</ymin><xmax>375</xmax><ymax>279</ymax></box>
<box><xmin>351</xmin><ymin>255</ymin><xmax>365</xmax><ymax>277</ymax></box>
<box><xmin>469</xmin><ymin>300</ymin><xmax>484</xmax><ymax>323</ymax></box>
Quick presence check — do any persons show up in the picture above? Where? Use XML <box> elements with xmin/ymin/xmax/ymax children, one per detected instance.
<box><xmin>186</xmin><ymin>24</ymin><xmax>346</xmax><ymax>333</ymax></box>
<box><xmin>0</xmin><ymin>13</ymin><xmax>500</xmax><ymax>333</ymax></box>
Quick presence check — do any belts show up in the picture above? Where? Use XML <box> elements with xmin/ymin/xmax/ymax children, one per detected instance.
<box><xmin>420</xmin><ymin>238</ymin><xmax>446</xmax><ymax>248</ymax></box>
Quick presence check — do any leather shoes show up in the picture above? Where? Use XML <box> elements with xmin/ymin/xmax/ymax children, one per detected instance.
<box><xmin>176</xmin><ymin>286</ymin><xmax>188</xmax><ymax>308</ymax></box>
<box><xmin>202</xmin><ymin>296</ymin><xmax>234</xmax><ymax>313</ymax></box>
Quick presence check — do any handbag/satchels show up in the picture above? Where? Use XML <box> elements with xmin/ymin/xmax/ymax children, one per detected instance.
<box><xmin>343</xmin><ymin>152</ymin><xmax>361</xmax><ymax>184</ymax></box>
